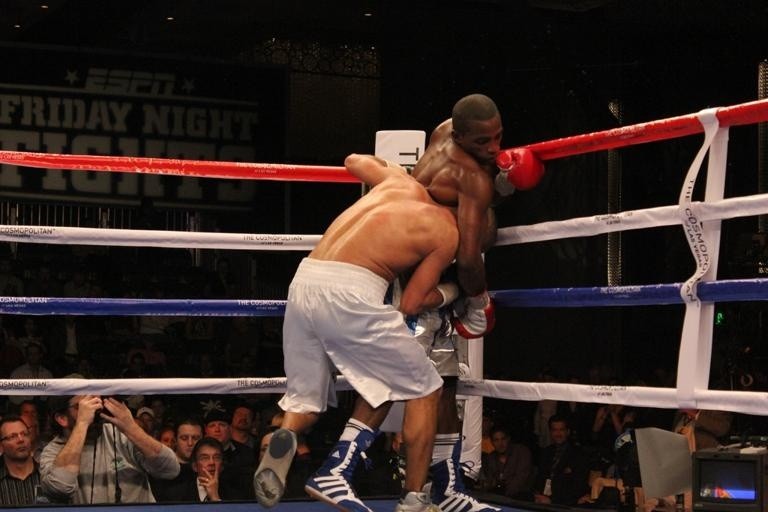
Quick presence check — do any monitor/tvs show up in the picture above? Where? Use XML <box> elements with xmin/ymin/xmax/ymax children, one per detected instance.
<box><xmin>691</xmin><ymin>444</ymin><xmax>768</xmax><ymax>512</ymax></box>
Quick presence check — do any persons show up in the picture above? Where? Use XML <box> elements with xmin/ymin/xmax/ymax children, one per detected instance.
<box><xmin>305</xmin><ymin>94</ymin><xmax>546</xmax><ymax>510</ymax></box>
<box><xmin>253</xmin><ymin>152</ymin><xmax>463</xmax><ymax>512</ymax></box>
<box><xmin>0</xmin><ymin>258</ymin><xmax>732</xmax><ymax>512</ymax></box>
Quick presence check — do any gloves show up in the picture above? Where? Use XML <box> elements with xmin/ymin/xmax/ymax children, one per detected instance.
<box><xmin>494</xmin><ymin>147</ymin><xmax>545</xmax><ymax>197</ymax></box>
<box><xmin>452</xmin><ymin>297</ymin><xmax>495</xmax><ymax>339</ymax></box>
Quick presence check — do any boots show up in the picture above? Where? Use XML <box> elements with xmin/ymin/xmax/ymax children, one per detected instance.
<box><xmin>431</xmin><ymin>440</ymin><xmax>503</xmax><ymax>511</ymax></box>
<box><xmin>304</xmin><ymin>429</ymin><xmax>372</xmax><ymax>512</ymax></box>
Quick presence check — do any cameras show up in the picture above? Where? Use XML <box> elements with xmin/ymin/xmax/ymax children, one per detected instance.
<box><xmin>94</xmin><ymin>395</ymin><xmax>126</xmax><ymax>423</ymax></box>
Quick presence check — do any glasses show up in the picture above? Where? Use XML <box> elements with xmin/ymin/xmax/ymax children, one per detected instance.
<box><xmin>1</xmin><ymin>432</ymin><xmax>29</xmax><ymax>440</ymax></box>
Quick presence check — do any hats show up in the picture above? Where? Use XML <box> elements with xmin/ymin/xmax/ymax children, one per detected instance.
<box><xmin>204</xmin><ymin>408</ymin><xmax>231</xmax><ymax>424</ymax></box>
<box><xmin>136</xmin><ymin>407</ymin><xmax>155</xmax><ymax>418</ymax></box>
<box><xmin>46</xmin><ymin>373</ymin><xmax>85</xmax><ymax>423</ymax></box>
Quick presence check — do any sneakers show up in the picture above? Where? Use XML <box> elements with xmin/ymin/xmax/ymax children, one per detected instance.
<box><xmin>253</xmin><ymin>428</ymin><xmax>298</xmax><ymax>507</ymax></box>
<box><xmin>396</xmin><ymin>481</ymin><xmax>442</xmax><ymax>512</ymax></box>
